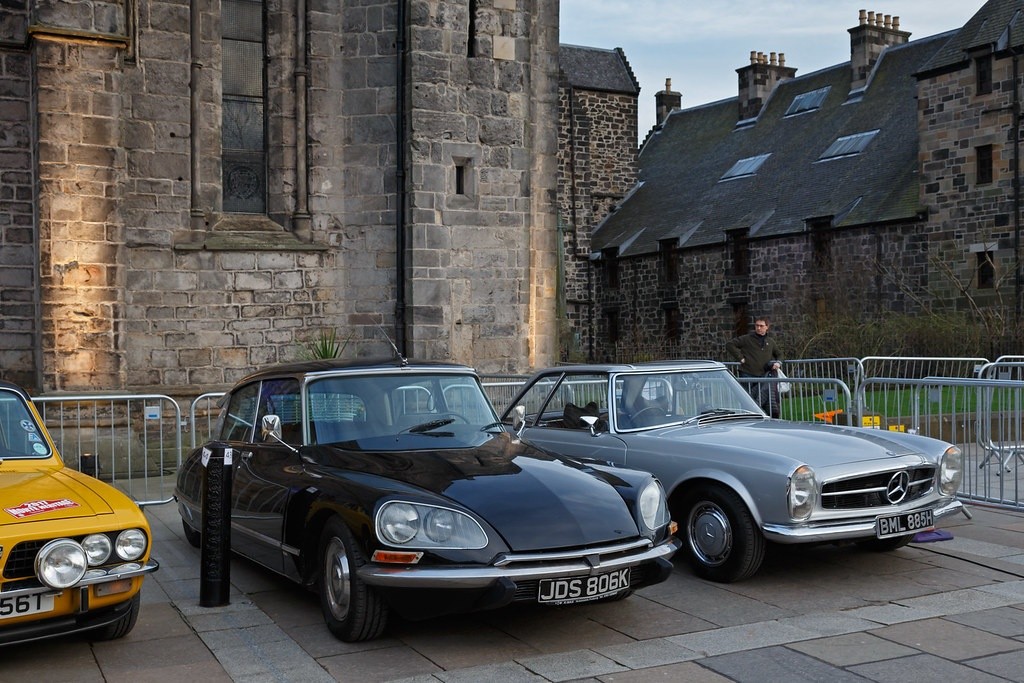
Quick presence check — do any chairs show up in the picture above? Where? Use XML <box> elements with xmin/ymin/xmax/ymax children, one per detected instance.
<box><xmin>634</xmin><ymin>396</ymin><xmax>670</xmax><ymax>423</ymax></box>
<box><xmin>564</xmin><ymin>402</ymin><xmax>599</xmax><ymax>430</ymax></box>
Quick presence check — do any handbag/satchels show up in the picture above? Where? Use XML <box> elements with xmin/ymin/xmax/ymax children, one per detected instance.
<box><xmin>776</xmin><ymin>367</ymin><xmax>792</xmax><ymax>393</ymax></box>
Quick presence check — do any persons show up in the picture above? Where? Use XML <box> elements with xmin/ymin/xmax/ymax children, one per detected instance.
<box><xmin>726</xmin><ymin>317</ymin><xmax>785</xmax><ymax>419</ymax></box>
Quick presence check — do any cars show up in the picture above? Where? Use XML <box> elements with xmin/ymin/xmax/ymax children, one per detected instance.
<box><xmin>499</xmin><ymin>360</ymin><xmax>963</xmax><ymax>584</ymax></box>
<box><xmin>0</xmin><ymin>381</ymin><xmax>160</xmax><ymax>648</ymax></box>
<box><xmin>173</xmin><ymin>358</ymin><xmax>682</xmax><ymax>643</ymax></box>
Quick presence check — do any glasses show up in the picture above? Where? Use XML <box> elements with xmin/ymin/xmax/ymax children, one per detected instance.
<box><xmin>755</xmin><ymin>324</ymin><xmax>766</xmax><ymax>327</ymax></box>
